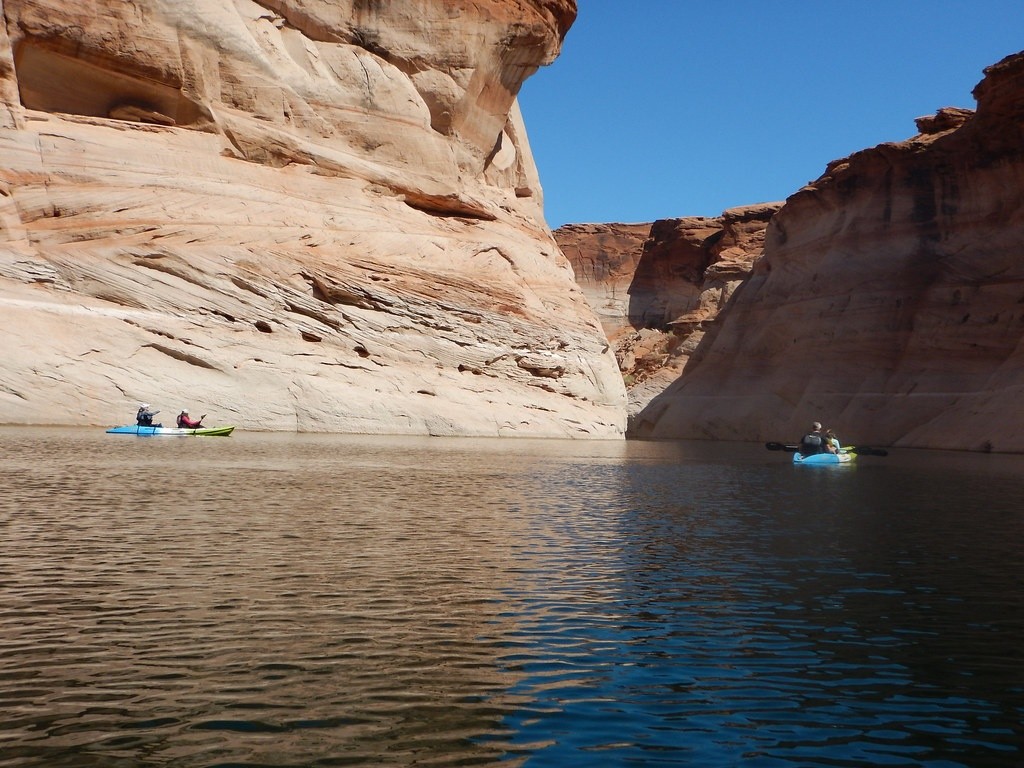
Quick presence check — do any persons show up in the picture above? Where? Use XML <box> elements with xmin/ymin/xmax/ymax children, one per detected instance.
<box><xmin>826</xmin><ymin>430</ymin><xmax>846</xmax><ymax>454</ymax></box>
<box><xmin>180</xmin><ymin>409</ymin><xmax>216</xmax><ymax>428</ymax></box>
<box><xmin>799</xmin><ymin>422</ymin><xmax>835</xmax><ymax>456</ymax></box>
<box><xmin>138</xmin><ymin>403</ymin><xmax>163</xmax><ymax>428</ymax></box>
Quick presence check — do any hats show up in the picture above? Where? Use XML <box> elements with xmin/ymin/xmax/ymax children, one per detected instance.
<box><xmin>140</xmin><ymin>402</ymin><xmax>150</xmax><ymax>409</ymax></box>
<box><xmin>181</xmin><ymin>410</ymin><xmax>188</xmax><ymax>415</ymax></box>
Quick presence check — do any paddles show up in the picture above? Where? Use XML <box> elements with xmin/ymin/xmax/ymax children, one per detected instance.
<box><xmin>200</xmin><ymin>413</ymin><xmax>207</xmax><ymax>422</ymax></box>
<box><xmin>765</xmin><ymin>441</ymin><xmax>889</xmax><ymax>457</ymax></box>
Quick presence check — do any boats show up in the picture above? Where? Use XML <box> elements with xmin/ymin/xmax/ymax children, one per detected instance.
<box><xmin>105</xmin><ymin>422</ymin><xmax>236</xmax><ymax>437</ymax></box>
<box><xmin>792</xmin><ymin>445</ymin><xmax>858</xmax><ymax>464</ymax></box>
